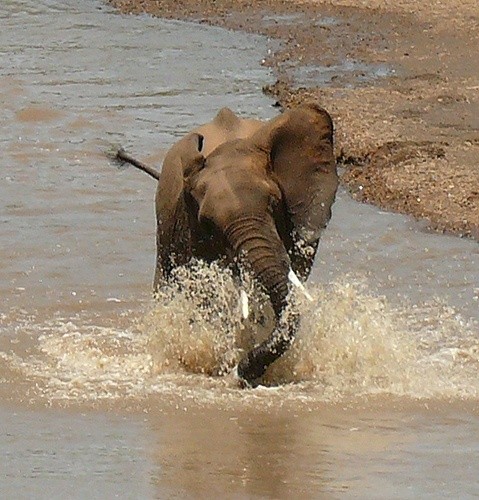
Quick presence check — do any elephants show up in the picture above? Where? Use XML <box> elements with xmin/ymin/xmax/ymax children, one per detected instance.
<box><xmin>154</xmin><ymin>103</ymin><xmax>340</xmax><ymax>386</ymax></box>
